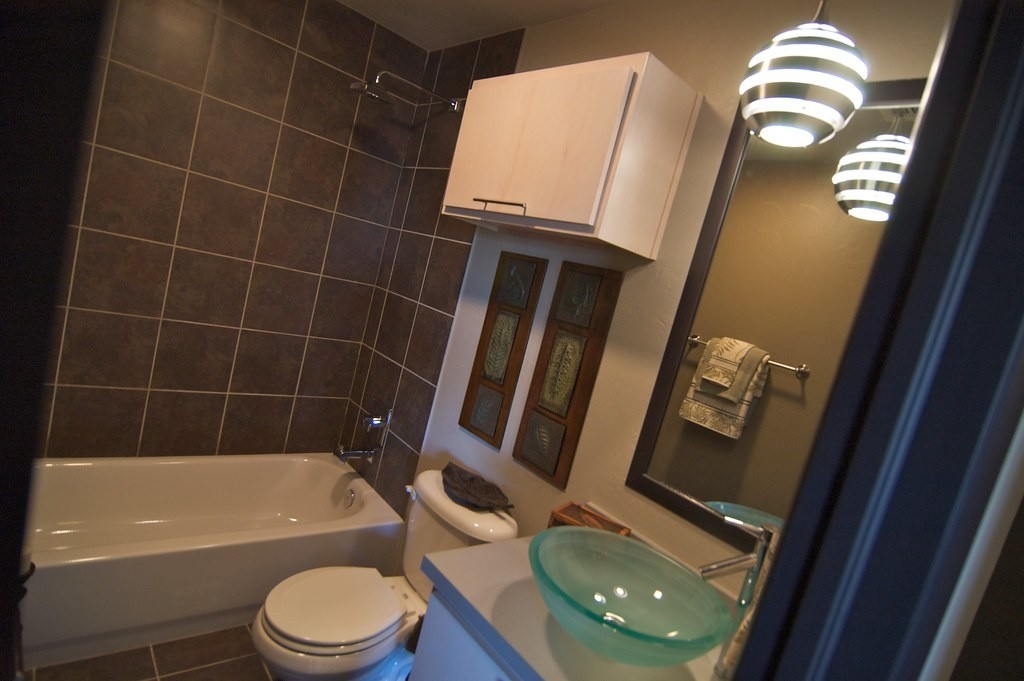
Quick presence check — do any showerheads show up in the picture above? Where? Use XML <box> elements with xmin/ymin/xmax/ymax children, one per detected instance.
<box><xmin>349</xmin><ymin>80</ymin><xmax>398</xmax><ymax>107</ymax></box>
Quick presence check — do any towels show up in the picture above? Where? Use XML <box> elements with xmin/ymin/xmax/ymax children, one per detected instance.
<box><xmin>694</xmin><ymin>338</ymin><xmax>769</xmax><ymax>405</ymax></box>
<box><xmin>701</xmin><ymin>337</ymin><xmax>756</xmax><ymax>389</ymax></box>
<box><xmin>676</xmin><ymin>338</ymin><xmax>771</xmax><ymax>441</ymax></box>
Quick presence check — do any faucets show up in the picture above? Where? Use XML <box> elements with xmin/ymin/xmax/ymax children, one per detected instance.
<box><xmin>335</xmin><ymin>438</ymin><xmax>380</xmax><ymax>470</ymax></box>
<box><xmin>699</xmin><ymin>512</ymin><xmax>773</xmax><ymax>680</ymax></box>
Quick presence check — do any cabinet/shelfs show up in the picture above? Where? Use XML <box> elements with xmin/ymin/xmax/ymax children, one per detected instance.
<box><xmin>441</xmin><ymin>50</ymin><xmax>705</xmax><ymax>264</ymax></box>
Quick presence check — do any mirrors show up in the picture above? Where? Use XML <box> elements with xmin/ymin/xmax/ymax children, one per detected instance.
<box><xmin>623</xmin><ymin>77</ymin><xmax>930</xmax><ymax>555</ymax></box>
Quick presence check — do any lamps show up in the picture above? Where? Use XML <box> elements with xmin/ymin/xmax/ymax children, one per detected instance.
<box><xmin>832</xmin><ymin>108</ymin><xmax>914</xmax><ymax>229</ymax></box>
<box><xmin>735</xmin><ymin>1</ymin><xmax>871</xmax><ymax>150</ymax></box>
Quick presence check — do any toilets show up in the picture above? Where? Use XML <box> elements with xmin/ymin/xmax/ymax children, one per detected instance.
<box><xmin>250</xmin><ymin>465</ymin><xmax>522</xmax><ymax>681</ymax></box>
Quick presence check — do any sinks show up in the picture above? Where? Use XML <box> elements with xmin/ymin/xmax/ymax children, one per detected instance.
<box><xmin>526</xmin><ymin>523</ymin><xmax>738</xmax><ymax>672</ymax></box>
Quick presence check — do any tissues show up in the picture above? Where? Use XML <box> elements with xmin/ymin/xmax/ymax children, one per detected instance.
<box><xmin>545</xmin><ymin>501</ymin><xmax>631</xmax><ymax>549</ymax></box>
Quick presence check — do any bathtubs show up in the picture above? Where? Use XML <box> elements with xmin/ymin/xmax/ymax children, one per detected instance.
<box><xmin>20</xmin><ymin>452</ymin><xmax>407</xmax><ymax>681</ymax></box>
<box><xmin>702</xmin><ymin>497</ymin><xmax>781</xmax><ymax>538</ymax></box>
